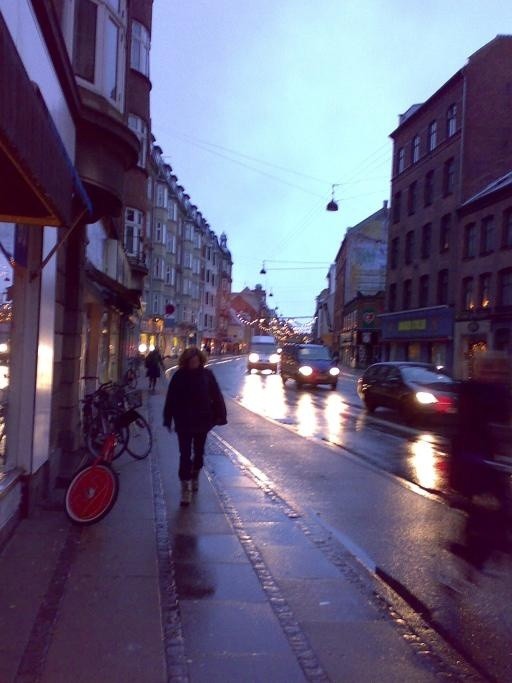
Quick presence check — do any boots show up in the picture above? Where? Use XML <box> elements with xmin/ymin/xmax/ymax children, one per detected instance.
<box><xmin>179</xmin><ymin>480</ymin><xmax>193</xmax><ymax>504</ymax></box>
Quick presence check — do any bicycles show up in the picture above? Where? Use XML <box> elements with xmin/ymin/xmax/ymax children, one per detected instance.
<box><xmin>63</xmin><ymin>361</ymin><xmax>152</xmax><ymax>526</ymax></box>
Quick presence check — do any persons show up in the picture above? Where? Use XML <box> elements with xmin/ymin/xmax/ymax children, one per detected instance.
<box><xmin>144</xmin><ymin>345</ymin><xmax>165</xmax><ymax>390</ymax></box>
<box><xmin>204</xmin><ymin>343</ymin><xmax>225</xmax><ymax>358</ymax></box>
<box><xmin>163</xmin><ymin>348</ymin><xmax>227</xmax><ymax>505</ymax></box>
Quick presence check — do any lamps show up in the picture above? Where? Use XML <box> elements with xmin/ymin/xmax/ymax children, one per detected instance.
<box><xmin>260</xmin><ymin>261</ymin><xmax>266</xmax><ymax>273</ymax></box>
<box><xmin>327</xmin><ymin>184</ymin><xmax>338</xmax><ymax>210</ymax></box>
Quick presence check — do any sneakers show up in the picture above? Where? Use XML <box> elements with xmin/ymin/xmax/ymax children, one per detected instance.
<box><xmin>192</xmin><ymin>480</ymin><xmax>199</xmax><ymax>492</ymax></box>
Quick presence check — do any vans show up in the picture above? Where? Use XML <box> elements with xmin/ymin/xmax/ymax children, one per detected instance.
<box><xmin>247</xmin><ymin>335</ymin><xmax>278</xmax><ymax>373</ymax></box>
<box><xmin>280</xmin><ymin>342</ymin><xmax>339</xmax><ymax>393</ymax></box>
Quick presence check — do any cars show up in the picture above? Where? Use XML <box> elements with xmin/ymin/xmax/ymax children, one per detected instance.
<box><xmin>356</xmin><ymin>361</ymin><xmax>478</xmax><ymax>424</ymax></box>
